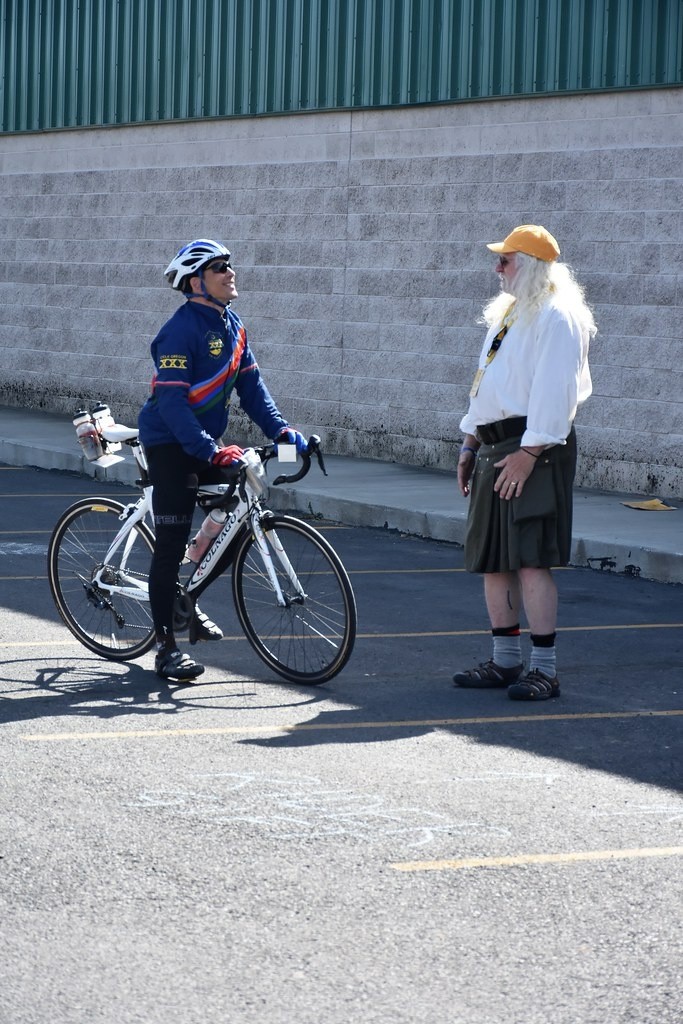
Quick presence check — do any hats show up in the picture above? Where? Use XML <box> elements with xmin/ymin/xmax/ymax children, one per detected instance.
<box><xmin>487</xmin><ymin>224</ymin><xmax>562</xmax><ymax>262</ymax></box>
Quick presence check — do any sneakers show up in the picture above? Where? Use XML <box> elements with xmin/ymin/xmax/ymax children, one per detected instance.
<box><xmin>155</xmin><ymin>646</ymin><xmax>205</xmax><ymax>678</ymax></box>
<box><xmin>184</xmin><ymin>614</ymin><xmax>223</xmax><ymax>644</ymax></box>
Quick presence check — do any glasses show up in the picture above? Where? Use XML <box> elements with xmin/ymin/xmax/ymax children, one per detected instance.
<box><xmin>205</xmin><ymin>262</ymin><xmax>232</xmax><ymax>274</ymax></box>
<box><xmin>499</xmin><ymin>256</ymin><xmax>516</xmax><ymax>266</ymax></box>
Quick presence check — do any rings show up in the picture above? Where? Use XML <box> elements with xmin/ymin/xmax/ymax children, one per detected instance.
<box><xmin>511</xmin><ymin>482</ymin><xmax>517</xmax><ymax>485</ymax></box>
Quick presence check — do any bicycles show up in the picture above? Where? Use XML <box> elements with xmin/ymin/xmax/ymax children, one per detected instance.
<box><xmin>47</xmin><ymin>399</ymin><xmax>358</xmax><ymax>687</ymax></box>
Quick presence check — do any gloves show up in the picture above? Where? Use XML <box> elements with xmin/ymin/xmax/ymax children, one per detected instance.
<box><xmin>272</xmin><ymin>428</ymin><xmax>309</xmax><ymax>454</ymax></box>
<box><xmin>209</xmin><ymin>445</ymin><xmax>250</xmax><ymax>466</ymax></box>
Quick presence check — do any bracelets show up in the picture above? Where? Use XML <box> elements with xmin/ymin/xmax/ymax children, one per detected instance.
<box><xmin>459</xmin><ymin>447</ymin><xmax>477</xmax><ymax>456</ymax></box>
<box><xmin>520</xmin><ymin>447</ymin><xmax>539</xmax><ymax>459</ymax></box>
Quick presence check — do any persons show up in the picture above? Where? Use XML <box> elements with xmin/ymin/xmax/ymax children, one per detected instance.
<box><xmin>454</xmin><ymin>224</ymin><xmax>597</xmax><ymax>700</ymax></box>
<box><xmin>138</xmin><ymin>239</ymin><xmax>309</xmax><ymax>681</ymax></box>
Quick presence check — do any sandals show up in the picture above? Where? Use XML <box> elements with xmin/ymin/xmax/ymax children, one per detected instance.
<box><xmin>508</xmin><ymin>666</ymin><xmax>561</xmax><ymax>700</ymax></box>
<box><xmin>452</xmin><ymin>659</ymin><xmax>526</xmax><ymax>687</ymax></box>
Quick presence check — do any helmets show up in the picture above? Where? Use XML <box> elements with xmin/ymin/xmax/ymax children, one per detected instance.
<box><xmin>163</xmin><ymin>239</ymin><xmax>231</xmax><ymax>290</ymax></box>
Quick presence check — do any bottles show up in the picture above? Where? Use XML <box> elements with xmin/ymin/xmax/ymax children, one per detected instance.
<box><xmin>188</xmin><ymin>506</ymin><xmax>227</xmax><ymax>564</ymax></box>
<box><xmin>72</xmin><ymin>408</ymin><xmax>103</xmax><ymax>461</ymax></box>
<box><xmin>178</xmin><ymin>544</ymin><xmax>190</xmax><ymax>565</ymax></box>
<box><xmin>92</xmin><ymin>400</ymin><xmax>122</xmax><ymax>454</ymax></box>
